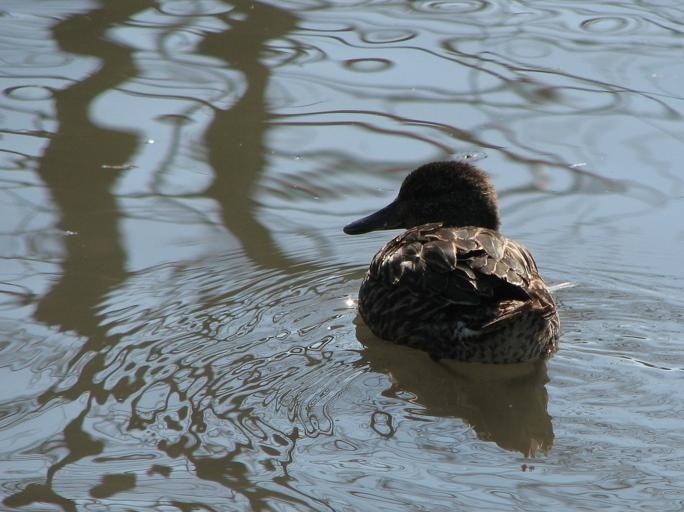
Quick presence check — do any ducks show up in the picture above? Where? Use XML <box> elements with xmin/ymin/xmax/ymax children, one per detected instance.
<box><xmin>343</xmin><ymin>159</ymin><xmax>561</xmax><ymax>364</ymax></box>
